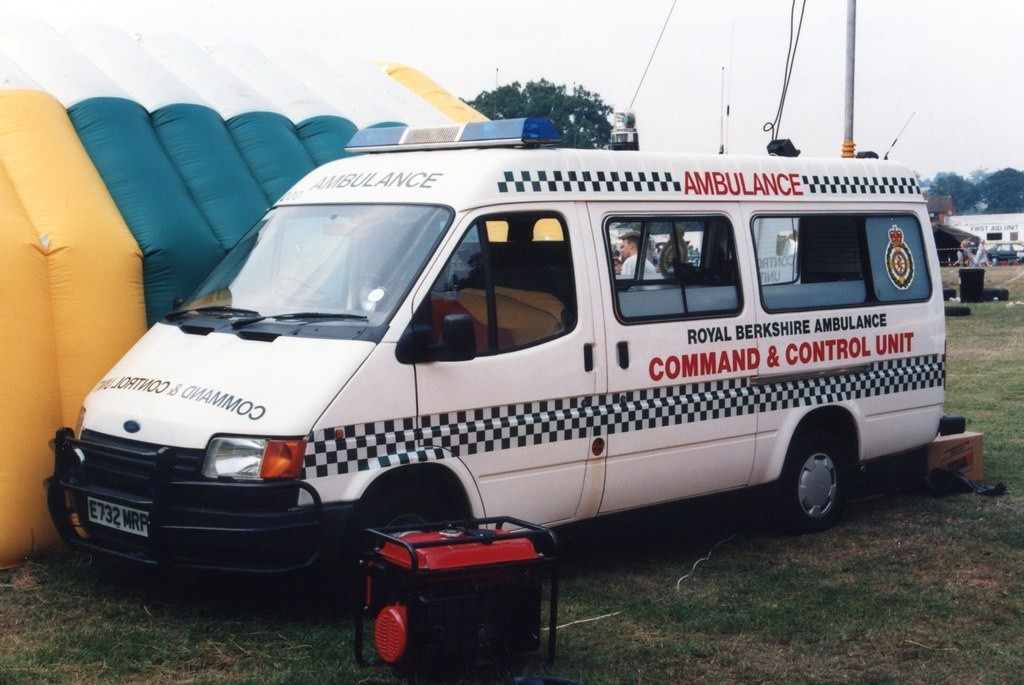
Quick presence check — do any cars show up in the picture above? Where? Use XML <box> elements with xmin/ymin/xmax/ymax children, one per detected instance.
<box><xmin>984</xmin><ymin>242</ymin><xmax>1024</xmax><ymax>266</ymax></box>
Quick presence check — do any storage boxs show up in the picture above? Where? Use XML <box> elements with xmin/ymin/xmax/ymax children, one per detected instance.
<box><xmin>914</xmin><ymin>432</ymin><xmax>984</xmax><ymax>482</ymax></box>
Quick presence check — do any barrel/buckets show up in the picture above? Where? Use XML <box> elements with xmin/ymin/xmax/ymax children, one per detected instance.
<box><xmin>959</xmin><ymin>268</ymin><xmax>985</xmax><ymax>303</ymax></box>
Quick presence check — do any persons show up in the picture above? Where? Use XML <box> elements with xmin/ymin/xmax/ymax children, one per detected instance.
<box><xmin>953</xmin><ymin>238</ymin><xmax>972</xmax><ymax>267</ymax></box>
<box><xmin>973</xmin><ymin>241</ymin><xmax>992</xmax><ymax>267</ymax></box>
<box><xmin>612</xmin><ymin>241</ymin><xmax>700</xmax><ymax>273</ymax></box>
<box><xmin>619</xmin><ymin>232</ymin><xmax>657</xmax><ymax>276</ymax></box>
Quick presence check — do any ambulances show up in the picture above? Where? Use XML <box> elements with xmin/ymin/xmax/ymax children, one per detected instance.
<box><xmin>46</xmin><ymin>114</ymin><xmax>968</xmax><ymax>603</ymax></box>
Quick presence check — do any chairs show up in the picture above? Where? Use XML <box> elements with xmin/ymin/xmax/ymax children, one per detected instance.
<box><xmin>492</xmin><ymin>241</ymin><xmax>569</xmax><ymax>301</ymax></box>
